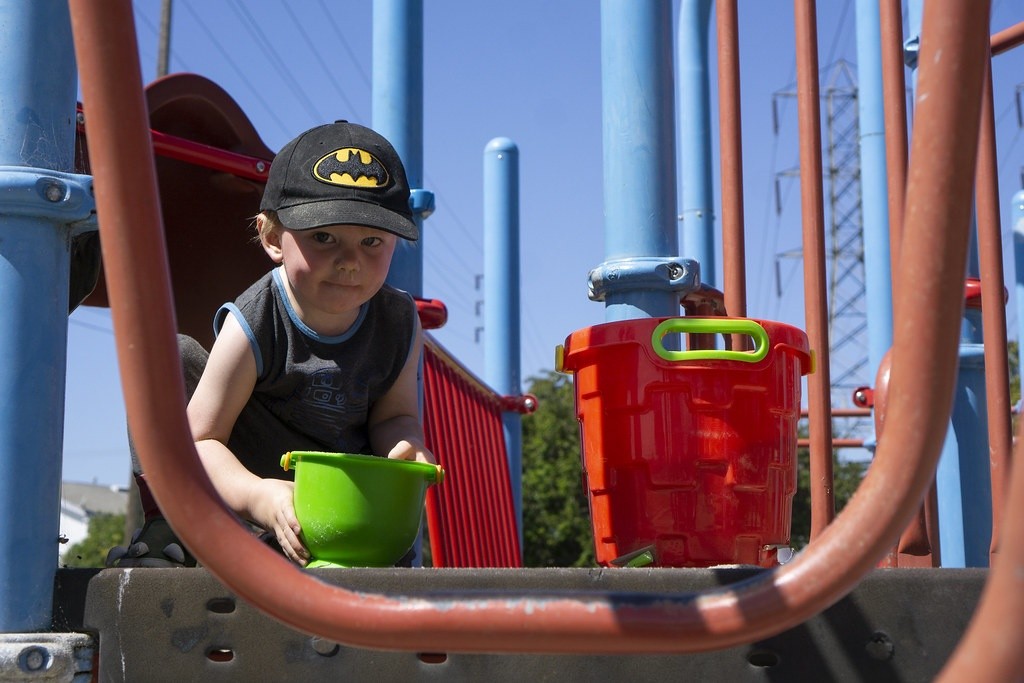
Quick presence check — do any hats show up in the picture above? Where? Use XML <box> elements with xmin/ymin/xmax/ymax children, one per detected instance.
<box><xmin>260</xmin><ymin>120</ymin><xmax>419</xmax><ymax>241</ymax></box>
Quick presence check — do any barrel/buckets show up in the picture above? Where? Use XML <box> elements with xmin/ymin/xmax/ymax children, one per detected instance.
<box><xmin>555</xmin><ymin>316</ymin><xmax>820</xmax><ymax>566</ymax></box>
<box><xmin>280</xmin><ymin>448</ymin><xmax>445</xmax><ymax>568</ymax></box>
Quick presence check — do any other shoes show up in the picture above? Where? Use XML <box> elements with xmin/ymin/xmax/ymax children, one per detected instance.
<box><xmin>105</xmin><ymin>518</ymin><xmax>198</xmax><ymax>569</ymax></box>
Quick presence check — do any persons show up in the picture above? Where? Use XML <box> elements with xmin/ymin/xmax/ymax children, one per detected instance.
<box><xmin>110</xmin><ymin>120</ymin><xmax>436</xmax><ymax>568</ymax></box>
